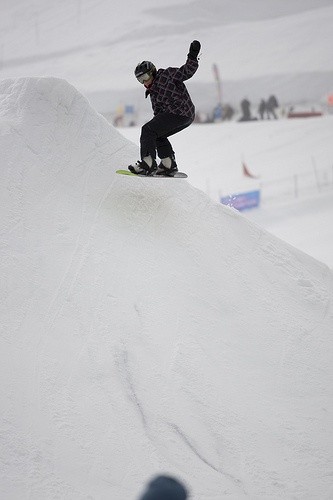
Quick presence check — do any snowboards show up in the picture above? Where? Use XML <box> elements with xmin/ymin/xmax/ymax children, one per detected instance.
<box><xmin>115</xmin><ymin>170</ymin><xmax>188</xmax><ymax>178</ymax></box>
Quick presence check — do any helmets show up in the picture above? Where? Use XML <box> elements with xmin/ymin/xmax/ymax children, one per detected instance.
<box><xmin>134</xmin><ymin>61</ymin><xmax>154</xmax><ymax>77</ymax></box>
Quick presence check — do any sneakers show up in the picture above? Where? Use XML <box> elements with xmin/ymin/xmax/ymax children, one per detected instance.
<box><xmin>156</xmin><ymin>159</ymin><xmax>179</xmax><ymax>178</ymax></box>
<box><xmin>128</xmin><ymin>157</ymin><xmax>159</xmax><ymax>176</ymax></box>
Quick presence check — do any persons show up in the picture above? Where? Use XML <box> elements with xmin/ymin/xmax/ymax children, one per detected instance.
<box><xmin>259</xmin><ymin>96</ymin><xmax>278</xmax><ymax>119</ymax></box>
<box><xmin>241</xmin><ymin>99</ymin><xmax>250</xmax><ymax>119</ymax></box>
<box><xmin>128</xmin><ymin>40</ymin><xmax>201</xmax><ymax>177</ymax></box>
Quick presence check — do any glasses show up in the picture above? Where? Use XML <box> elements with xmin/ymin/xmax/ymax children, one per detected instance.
<box><xmin>137</xmin><ymin>66</ymin><xmax>156</xmax><ymax>84</ymax></box>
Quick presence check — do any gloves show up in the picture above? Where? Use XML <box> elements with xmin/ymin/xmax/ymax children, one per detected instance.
<box><xmin>187</xmin><ymin>40</ymin><xmax>201</xmax><ymax>61</ymax></box>
